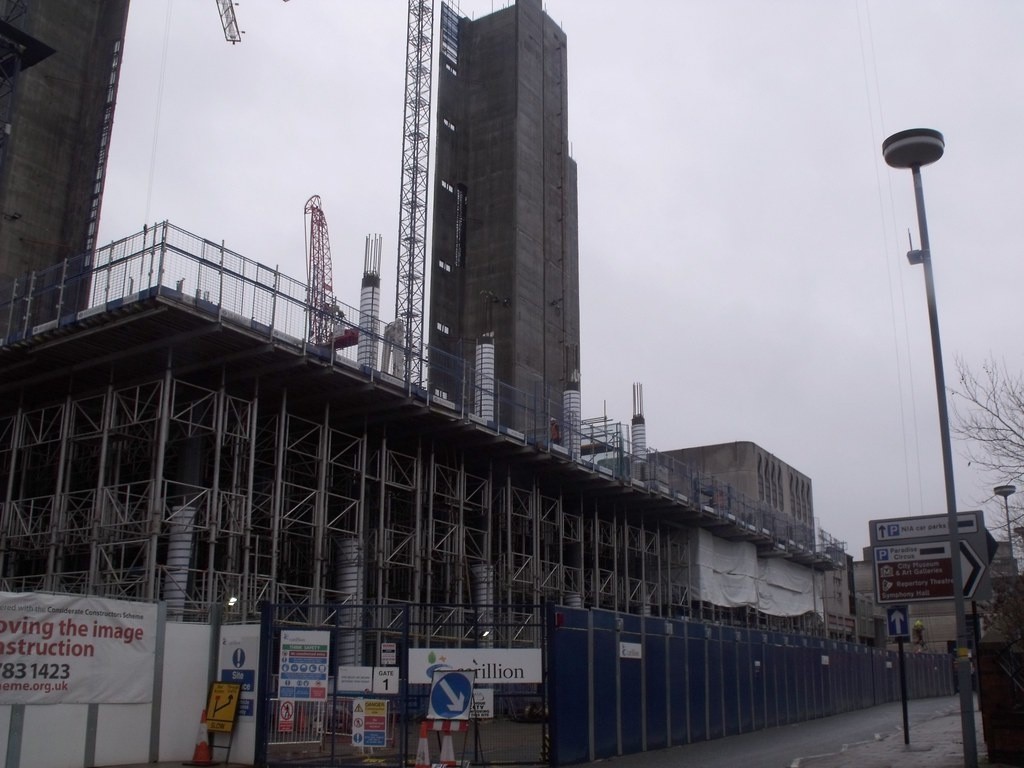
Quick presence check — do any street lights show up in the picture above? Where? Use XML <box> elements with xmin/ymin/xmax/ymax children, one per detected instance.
<box><xmin>881</xmin><ymin>128</ymin><xmax>981</xmax><ymax>763</ymax></box>
<box><xmin>994</xmin><ymin>484</ymin><xmax>1016</xmax><ymax>564</ymax></box>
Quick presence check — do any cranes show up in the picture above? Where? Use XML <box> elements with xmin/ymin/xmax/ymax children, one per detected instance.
<box><xmin>300</xmin><ymin>192</ymin><xmax>356</xmax><ymax>347</ymax></box>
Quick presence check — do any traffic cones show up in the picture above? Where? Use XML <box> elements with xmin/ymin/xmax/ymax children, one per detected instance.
<box><xmin>440</xmin><ymin>722</ymin><xmax>457</xmax><ymax>768</ymax></box>
<box><xmin>415</xmin><ymin>722</ymin><xmax>432</xmax><ymax>768</ymax></box>
<box><xmin>193</xmin><ymin>709</ymin><xmax>210</xmax><ymax>761</ymax></box>
